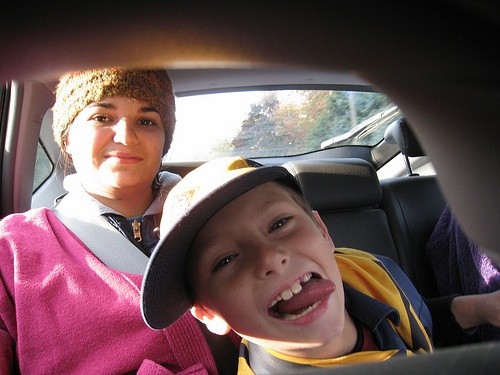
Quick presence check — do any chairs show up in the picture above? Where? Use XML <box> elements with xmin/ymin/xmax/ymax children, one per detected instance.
<box><xmin>379</xmin><ymin>175</ymin><xmax>446</xmax><ymax>320</ymax></box>
<box><xmin>281</xmin><ymin>157</ymin><xmax>396</xmax><ymax>264</ymax></box>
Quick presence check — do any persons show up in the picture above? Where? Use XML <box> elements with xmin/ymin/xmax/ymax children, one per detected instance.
<box><xmin>140</xmin><ymin>156</ymin><xmax>500</xmax><ymax>375</ymax></box>
<box><xmin>0</xmin><ymin>68</ymin><xmax>500</xmax><ymax>375</ymax></box>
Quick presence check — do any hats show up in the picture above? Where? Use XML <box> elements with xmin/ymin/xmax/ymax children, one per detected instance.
<box><xmin>140</xmin><ymin>157</ymin><xmax>304</xmax><ymax>332</ymax></box>
<box><xmin>52</xmin><ymin>68</ymin><xmax>176</xmax><ymax>158</ymax></box>
